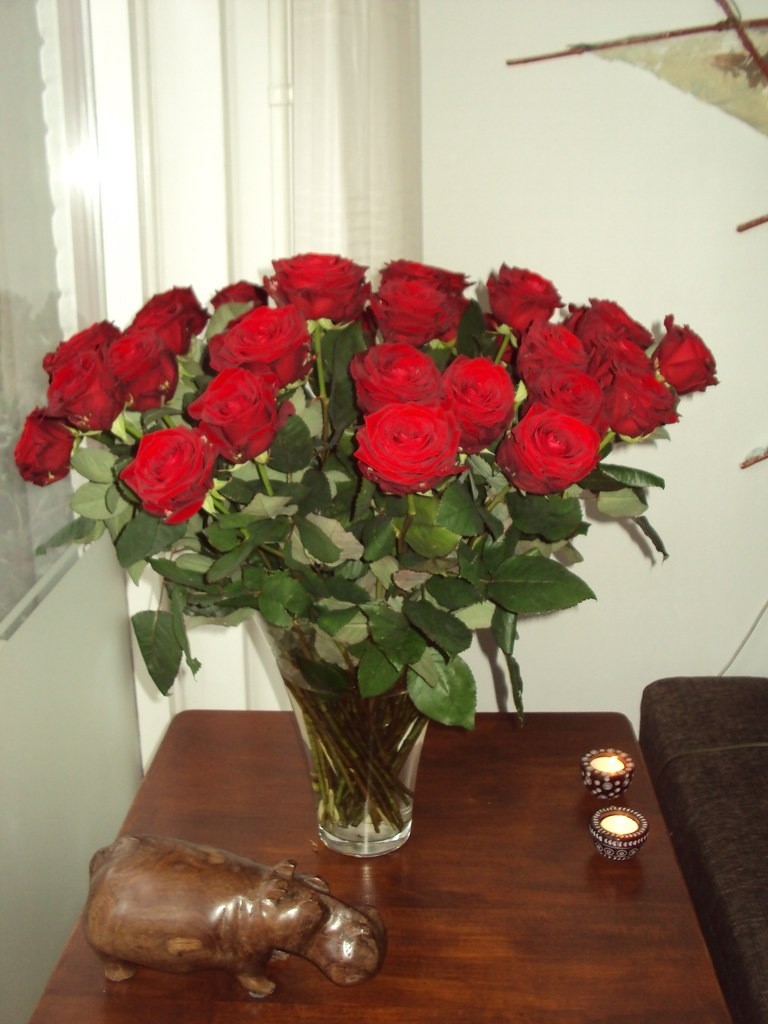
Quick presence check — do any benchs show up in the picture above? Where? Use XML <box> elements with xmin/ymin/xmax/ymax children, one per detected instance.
<box><xmin>639</xmin><ymin>674</ymin><xmax>768</xmax><ymax>1023</ymax></box>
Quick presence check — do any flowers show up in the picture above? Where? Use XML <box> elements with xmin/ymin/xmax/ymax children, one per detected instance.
<box><xmin>11</xmin><ymin>238</ymin><xmax>721</xmax><ymax>829</ymax></box>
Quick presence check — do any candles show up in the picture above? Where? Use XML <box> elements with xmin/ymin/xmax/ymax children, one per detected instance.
<box><xmin>599</xmin><ymin>814</ymin><xmax>639</xmax><ymax>834</ymax></box>
<box><xmin>588</xmin><ymin>754</ymin><xmax>628</xmax><ymax>773</ymax></box>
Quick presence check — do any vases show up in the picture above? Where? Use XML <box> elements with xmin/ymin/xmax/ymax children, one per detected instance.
<box><xmin>256</xmin><ymin>611</ymin><xmax>432</xmax><ymax>856</ymax></box>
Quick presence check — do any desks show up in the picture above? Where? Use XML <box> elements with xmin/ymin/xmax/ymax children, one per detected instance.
<box><xmin>30</xmin><ymin>710</ymin><xmax>732</xmax><ymax>1024</ymax></box>
